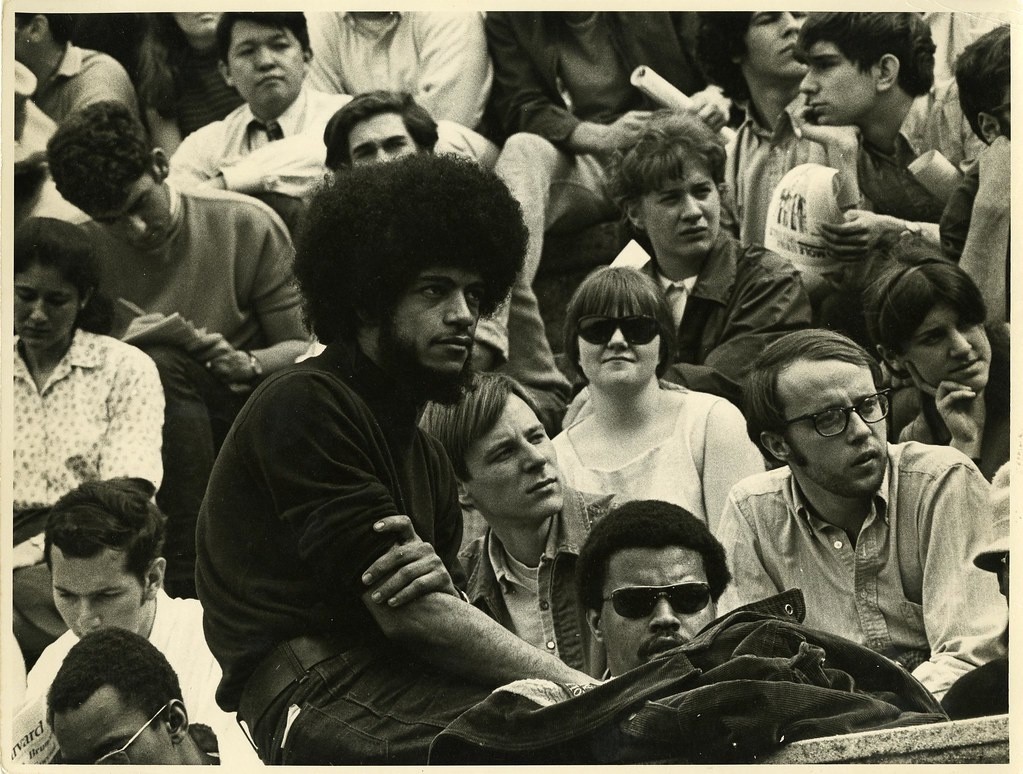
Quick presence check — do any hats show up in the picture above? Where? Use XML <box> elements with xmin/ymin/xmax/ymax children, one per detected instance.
<box><xmin>974</xmin><ymin>460</ymin><xmax>1009</xmax><ymax>575</ymax></box>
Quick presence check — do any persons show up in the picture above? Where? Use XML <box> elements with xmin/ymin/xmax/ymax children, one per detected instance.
<box><xmin>15</xmin><ymin>11</ymin><xmax>1010</xmax><ymax>765</ymax></box>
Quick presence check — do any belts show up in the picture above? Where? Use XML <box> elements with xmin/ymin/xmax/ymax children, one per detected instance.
<box><xmin>240</xmin><ymin>628</ymin><xmax>360</xmax><ymax>746</ymax></box>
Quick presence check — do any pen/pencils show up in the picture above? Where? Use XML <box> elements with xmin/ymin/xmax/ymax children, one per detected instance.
<box><xmin>118</xmin><ymin>297</ymin><xmax>146</xmax><ymax>316</ymax></box>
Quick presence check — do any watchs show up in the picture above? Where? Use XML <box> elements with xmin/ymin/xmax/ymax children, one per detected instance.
<box><xmin>245</xmin><ymin>349</ymin><xmax>262</xmax><ymax>382</ymax></box>
<box><xmin>900</xmin><ymin>230</ymin><xmax>913</xmax><ymax>241</ymax></box>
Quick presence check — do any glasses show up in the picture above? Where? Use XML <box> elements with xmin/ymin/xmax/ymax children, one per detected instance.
<box><xmin>786</xmin><ymin>388</ymin><xmax>891</xmax><ymax>437</ymax></box>
<box><xmin>95</xmin><ymin>704</ymin><xmax>166</xmax><ymax>763</ymax></box>
<box><xmin>574</xmin><ymin>314</ymin><xmax>660</xmax><ymax>344</ymax></box>
<box><xmin>602</xmin><ymin>583</ymin><xmax>713</xmax><ymax>619</ymax></box>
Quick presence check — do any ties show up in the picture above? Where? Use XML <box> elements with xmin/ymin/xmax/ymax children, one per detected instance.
<box><xmin>265</xmin><ymin>123</ymin><xmax>284</xmax><ymax>143</ymax></box>
